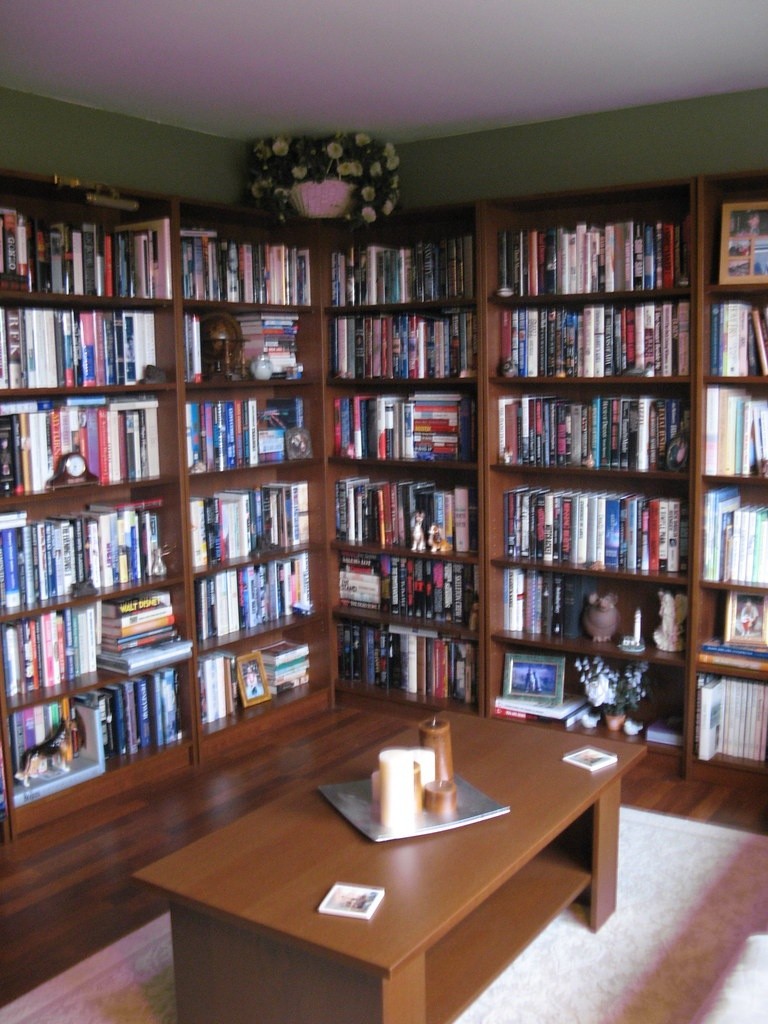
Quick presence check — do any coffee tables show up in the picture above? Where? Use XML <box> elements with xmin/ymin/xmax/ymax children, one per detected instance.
<box><xmin>130</xmin><ymin>709</ymin><xmax>650</xmax><ymax>1024</ymax></box>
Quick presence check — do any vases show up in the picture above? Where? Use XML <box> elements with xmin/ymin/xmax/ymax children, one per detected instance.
<box><xmin>605</xmin><ymin>714</ymin><xmax>626</xmax><ymax>731</ymax></box>
<box><xmin>290</xmin><ymin>180</ymin><xmax>357</xmax><ymax>219</ymax></box>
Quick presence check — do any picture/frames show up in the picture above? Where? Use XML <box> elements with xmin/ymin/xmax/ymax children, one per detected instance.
<box><xmin>236</xmin><ymin>651</ymin><xmax>272</xmax><ymax>709</ymax></box>
<box><xmin>723</xmin><ymin>590</ymin><xmax>768</xmax><ymax>645</ymax></box>
<box><xmin>718</xmin><ymin>201</ymin><xmax>768</xmax><ymax>285</ymax></box>
<box><xmin>502</xmin><ymin>651</ymin><xmax>566</xmax><ymax>706</ymax></box>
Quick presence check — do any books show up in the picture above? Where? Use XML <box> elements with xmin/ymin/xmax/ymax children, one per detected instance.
<box><xmin>503</xmin><ymin>568</ymin><xmax>598</xmax><ymax>637</ymax></box>
<box><xmin>181</xmin><ymin>222</ymin><xmax>311</xmax><ymax>307</ymax></box>
<box><xmin>644</xmin><ymin>713</ymin><xmax>684</xmax><ymax>747</ymax></box>
<box><xmin>498</xmin><ymin>392</ymin><xmax>690</xmax><ymax>571</ymax></box>
<box><xmin>495</xmin><ymin>692</ymin><xmax>593</xmax><ymax>728</ymax></box>
<box><xmin>197</xmin><ymin>641</ymin><xmax>310</xmax><ymax>725</ymax></box>
<box><xmin>191</xmin><ymin>480</ymin><xmax>310</xmax><ymax>567</ymax></box>
<box><xmin>333</xmin><ymin>390</ymin><xmax>480</xmax><ymax>552</ymax></box>
<box><xmin>0</xmin><ymin>207</ymin><xmax>172</xmax><ymax>300</ymax></box>
<box><xmin>337</xmin><ymin>550</ymin><xmax>478</xmax><ymax>704</ymax></box>
<box><xmin>186</xmin><ymin>396</ymin><xmax>303</xmax><ymax>474</ymax></box>
<box><xmin>0</xmin><ymin>306</ymin><xmax>156</xmax><ymax>389</ymax></box>
<box><xmin>499</xmin><ymin>216</ymin><xmax>690</xmax><ymax>378</ymax></box>
<box><xmin>183</xmin><ymin>312</ymin><xmax>304</xmax><ymax>384</ymax></box>
<box><xmin>0</xmin><ymin>396</ymin><xmax>193</xmax><ymax>808</ymax></box>
<box><xmin>194</xmin><ymin>551</ymin><xmax>312</xmax><ymax>642</ymax></box>
<box><xmin>696</xmin><ymin>296</ymin><xmax>768</xmax><ymax>761</ymax></box>
<box><xmin>329</xmin><ymin>235</ymin><xmax>478</xmax><ymax>380</ymax></box>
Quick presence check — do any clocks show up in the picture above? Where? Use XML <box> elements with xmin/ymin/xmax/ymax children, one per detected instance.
<box><xmin>45</xmin><ymin>452</ymin><xmax>100</xmax><ymax>492</ymax></box>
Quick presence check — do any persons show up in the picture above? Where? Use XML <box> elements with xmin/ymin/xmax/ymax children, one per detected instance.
<box><xmin>659</xmin><ymin>594</ymin><xmax>678</xmax><ymax>642</ymax></box>
<box><xmin>246</xmin><ymin>666</ymin><xmax>257</xmax><ymax>695</ymax></box>
<box><xmin>741</xmin><ymin>600</ymin><xmax>758</xmax><ymax>637</ymax></box>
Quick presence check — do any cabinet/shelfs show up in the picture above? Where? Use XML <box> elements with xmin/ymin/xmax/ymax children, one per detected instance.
<box><xmin>0</xmin><ymin>166</ymin><xmax>768</xmax><ymax>1009</ymax></box>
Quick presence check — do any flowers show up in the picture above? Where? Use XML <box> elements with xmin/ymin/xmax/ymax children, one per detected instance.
<box><xmin>575</xmin><ymin>655</ymin><xmax>650</xmax><ymax>716</ymax></box>
<box><xmin>245</xmin><ymin>126</ymin><xmax>401</xmax><ymax>223</ymax></box>
